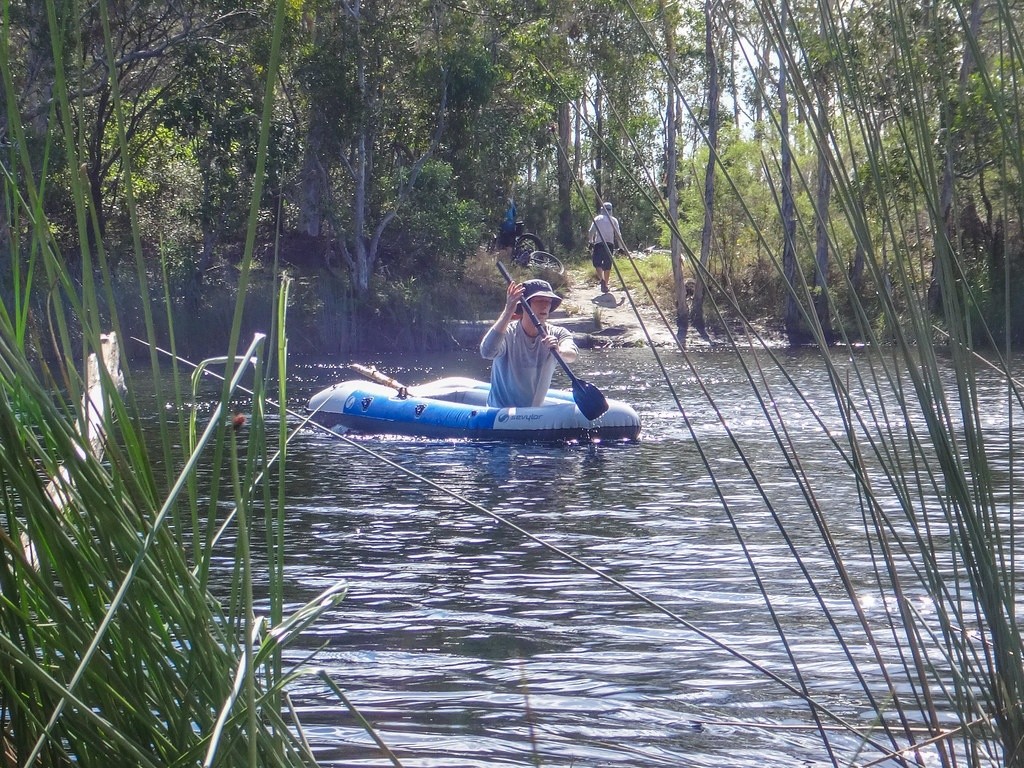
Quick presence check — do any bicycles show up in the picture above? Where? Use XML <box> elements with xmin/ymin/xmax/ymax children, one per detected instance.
<box><xmin>480</xmin><ymin>220</ymin><xmax>565</xmax><ymax>280</ymax></box>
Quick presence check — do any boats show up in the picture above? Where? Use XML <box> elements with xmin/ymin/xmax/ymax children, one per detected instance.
<box><xmin>305</xmin><ymin>374</ymin><xmax>643</xmax><ymax>446</ymax></box>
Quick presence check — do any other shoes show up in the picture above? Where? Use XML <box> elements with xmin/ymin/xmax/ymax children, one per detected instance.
<box><xmin>601</xmin><ymin>279</ymin><xmax>607</xmax><ymax>292</ymax></box>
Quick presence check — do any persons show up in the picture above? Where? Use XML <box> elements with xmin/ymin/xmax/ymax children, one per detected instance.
<box><xmin>480</xmin><ymin>280</ymin><xmax>578</xmax><ymax>408</ymax></box>
<box><xmin>501</xmin><ymin>197</ymin><xmax>517</xmax><ymax>248</ymax></box>
<box><xmin>588</xmin><ymin>202</ymin><xmax>624</xmax><ymax>293</ymax></box>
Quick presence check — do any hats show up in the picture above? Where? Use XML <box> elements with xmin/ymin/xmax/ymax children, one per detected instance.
<box><xmin>514</xmin><ymin>279</ymin><xmax>563</xmax><ymax>315</ymax></box>
<box><xmin>601</xmin><ymin>202</ymin><xmax>612</xmax><ymax>210</ymax></box>
